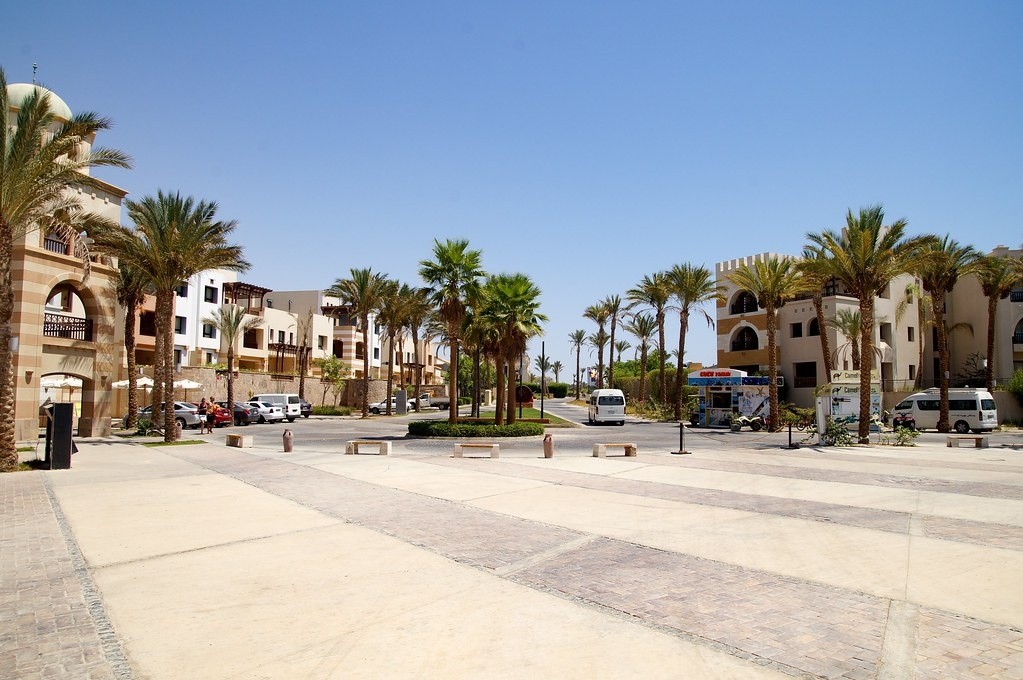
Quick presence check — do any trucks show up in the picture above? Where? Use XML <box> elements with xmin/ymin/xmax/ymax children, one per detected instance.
<box><xmin>407</xmin><ymin>393</ymin><xmax>450</xmax><ymax>410</ymax></box>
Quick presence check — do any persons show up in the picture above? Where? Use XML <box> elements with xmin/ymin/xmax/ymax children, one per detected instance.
<box><xmin>199</xmin><ymin>396</ymin><xmax>217</xmax><ymax>435</ymax></box>
<box><xmin>882</xmin><ymin>412</ymin><xmax>888</xmax><ymax>427</ymax></box>
<box><xmin>217</xmin><ymin>371</ymin><xmax>231</xmax><ymax>380</ymax></box>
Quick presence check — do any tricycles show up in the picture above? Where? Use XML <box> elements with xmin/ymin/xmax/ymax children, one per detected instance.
<box><xmin>892</xmin><ymin>412</ymin><xmax>915</xmax><ymax>433</ymax></box>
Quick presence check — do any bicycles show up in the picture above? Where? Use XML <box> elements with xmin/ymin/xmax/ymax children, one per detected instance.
<box><xmin>796</xmin><ymin>414</ymin><xmax>818</xmax><ymax>431</ymax></box>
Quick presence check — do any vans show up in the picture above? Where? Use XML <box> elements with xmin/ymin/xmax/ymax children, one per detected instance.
<box><xmin>586</xmin><ymin>388</ymin><xmax>627</xmax><ymax>426</ymax></box>
<box><xmin>247</xmin><ymin>393</ymin><xmax>301</xmax><ymax>422</ymax></box>
<box><xmin>889</xmin><ymin>387</ymin><xmax>998</xmax><ymax>434</ymax></box>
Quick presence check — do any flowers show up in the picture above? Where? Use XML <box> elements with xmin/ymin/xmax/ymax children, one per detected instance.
<box><xmin>216</xmin><ymin>372</ymin><xmax>223</xmax><ymax>381</ymax></box>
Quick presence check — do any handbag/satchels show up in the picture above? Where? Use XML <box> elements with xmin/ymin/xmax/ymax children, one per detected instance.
<box><xmin>207</xmin><ymin>414</ymin><xmax>216</xmax><ymax>421</ymax></box>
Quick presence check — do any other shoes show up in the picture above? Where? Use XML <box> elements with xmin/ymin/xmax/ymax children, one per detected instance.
<box><xmin>201</xmin><ymin>431</ymin><xmax>204</xmax><ymax>435</ymax></box>
<box><xmin>207</xmin><ymin>429</ymin><xmax>213</xmax><ymax>434</ymax></box>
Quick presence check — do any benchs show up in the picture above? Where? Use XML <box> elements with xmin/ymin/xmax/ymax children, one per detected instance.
<box><xmin>226</xmin><ymin>434</ymin><xmax>253</xmax><ymax>448</ymax></box>
<box><xmin>946</xmin><ymin>436</ymin><xmax>988</xmax><ymax>447</ymax></box>
<box><xmin>345</xmin><ymin>441</ymin><xmax>392</xmax><ymax>455</ymax></box>
<box><xmin>454</xmin><ymin>444</ymin><xmax>500</xmax><ymax>458</ymax></box>
<box><xmin>593</xmin><ymin>443</ymin><xmax>638</xmax><ymax>457</ymax></box>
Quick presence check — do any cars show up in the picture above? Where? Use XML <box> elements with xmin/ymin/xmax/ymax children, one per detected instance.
<box><xmin>190</xmin><ymin>402</ymin><xmax>233</xmax><ymax>428</ymax></box>
<box><xmin>244</xmin><ymin>401</ymin><xmax>285</xmax><ymax>424</ymax></box>
<box><xmin>122</xmin><ymin>400</ymin><xmax>208</xmax><ymax>430</ymax></box>
<box><xmin>213</xmin><ymin>400</ymin><xmax>260</xmax><ymax>426</ymax></box>
<box><xmin>369</xmin><ymin>396</ymin><xmax>412</xmax><ymax>414</ymax></box>
<box><xmin>300</xmin><ymin>398</ymin><xmax>314</xmax><ymax>419</ymax></box>
